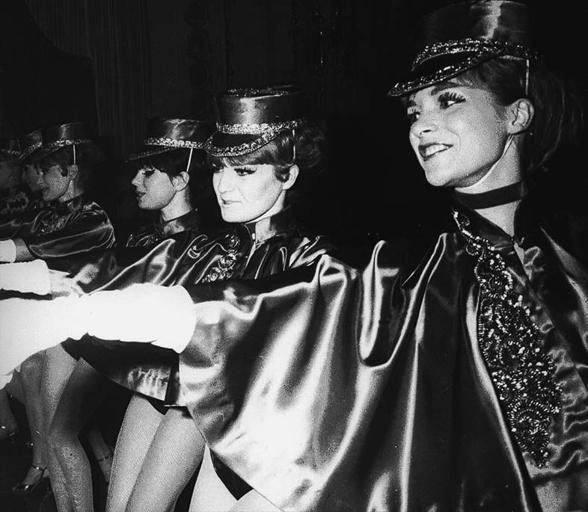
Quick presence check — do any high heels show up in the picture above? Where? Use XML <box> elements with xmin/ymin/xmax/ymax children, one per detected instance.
<box><xmin>14</xmin><ymin>465</ymin><xmax>51</xmax><ymax>495</ymax></box>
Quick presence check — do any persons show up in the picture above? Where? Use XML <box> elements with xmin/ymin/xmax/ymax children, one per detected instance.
<box><xmin>1</xmin><ymin>0</ymin><xmax>588</xmax><ymax>512</ymax></box>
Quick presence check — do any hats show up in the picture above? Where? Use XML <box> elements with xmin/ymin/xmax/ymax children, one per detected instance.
<box><xmin>127</xmin><ymin>117</ymin><xmax>210</xmax><ymax>162</ymax></box>
<box><xmin>2</xmin><ymin>119</ymin><xmax>87</xmax><ymax>164</ymax></box>
<box><xmin>387</xmin><ymin>1</ymin><xmax>542</xmax><ymax>99</ymax></box>
<box><xmin>202</xmin><ymin>86</ymin><xmax>305</xmax><ymax>153</ymax></box>
